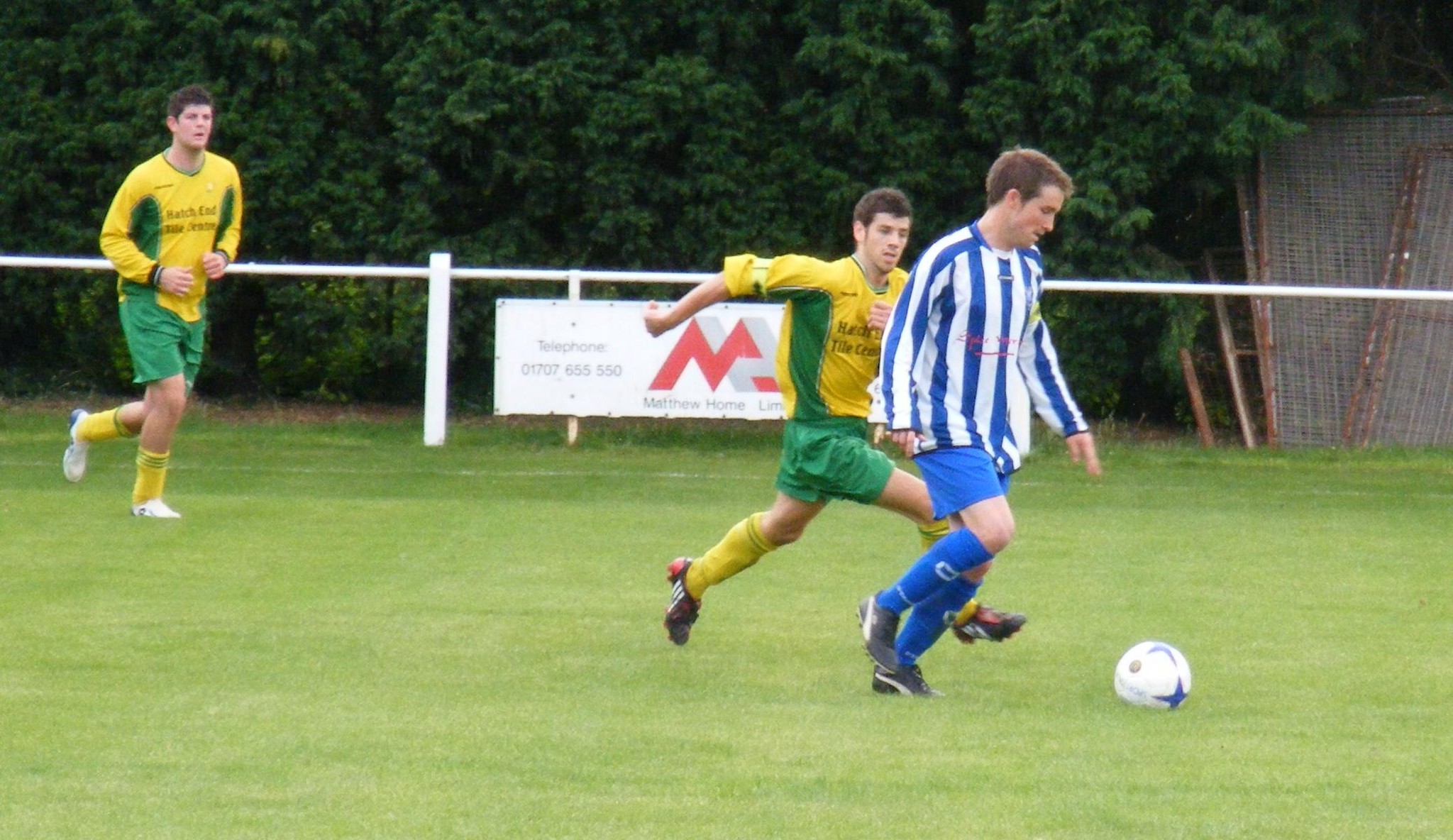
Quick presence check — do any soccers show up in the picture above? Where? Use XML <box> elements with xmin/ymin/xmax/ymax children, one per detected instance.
<box><xmin>1114</xmin><ymin>640</ymin><xmax>1192</xmax><ymax>709</ymax></box>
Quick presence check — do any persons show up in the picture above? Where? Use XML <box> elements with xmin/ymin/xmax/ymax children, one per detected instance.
<box><xmin>646</xmin><ymin>186</ymin><xmax>1027</xmax><ymax>649</ymax></box>
<box><xmin>852</xmin><ymin>141</ymin><xmax>1102</xmax><ymax>700</ymax></box>
<box><xmin>63</xmin><ymin>83</ymin><xmax>243</xmax><ymax>518</ymax></box>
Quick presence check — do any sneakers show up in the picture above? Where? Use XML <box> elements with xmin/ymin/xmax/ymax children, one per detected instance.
<box><xmin>856</xmin><ymin>590</ymin><xmax>899</xmax><ymax>675</ymax></box>
<box><xmin>872</xmin><ymin>662</ymin><xmax>945</xmax><ymax>701</ymax></box>
<box><xmin>664</xmin><ymin>558</ymin><xmax>702</xmax><ymax>645</ymax></box>
<box><xmin>134</xmin><ymin>500</ymin><xmax>180</xmax><ymax>518</ymax></box>
<box><xmin>63</xmin><ymin>409</ymin><xmax>89</xmax><ymax>482</ymax></box>
<box><xmin>952</xmin><ymin>602</ymin><xmax>1027</xmax><ymax>645</ymax></box>
